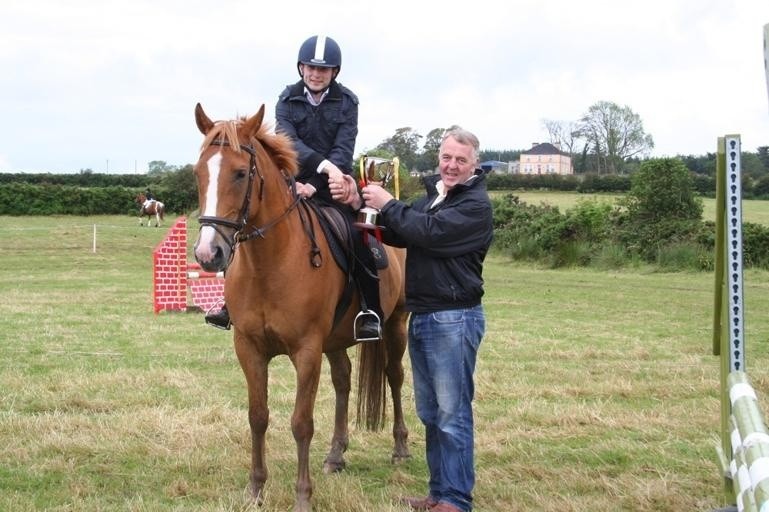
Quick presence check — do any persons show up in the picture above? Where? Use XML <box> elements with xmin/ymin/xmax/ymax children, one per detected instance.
<box><xmin>139</xmin><ymin>188</ymin><xmax>153</xmax><ymax>212</ymax></box>
<box><xmin>327</xmin><ymin>126</ymin><xmax>494</xmax><ymax>512</ymax></box>
<box><xmin>204</xmin><ymin>34</ymin><xmax>386</xmax><ymax>336</ymax></box>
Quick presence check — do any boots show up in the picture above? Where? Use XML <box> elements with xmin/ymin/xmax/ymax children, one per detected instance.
<box><xmin>349</xmin><ymin>246</ymin><xmax>386</xmax><ymax>337</ymax></box>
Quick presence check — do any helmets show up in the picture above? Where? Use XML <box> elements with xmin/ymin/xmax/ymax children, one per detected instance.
<box><xmin>295</xmin><ymin>34</ymin><xmax>344</xmax><ymax>69</ymax></box>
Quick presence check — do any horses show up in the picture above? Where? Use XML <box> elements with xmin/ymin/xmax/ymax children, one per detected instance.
<box><xmin>135</xmin><ymin>192</ymin><xmax>165</xmax><ymax>228</ymax></box>
<box><xmin>193</xmin><ymin>101</ymin><xmax>411</xmax><ymax>512</ymax></box>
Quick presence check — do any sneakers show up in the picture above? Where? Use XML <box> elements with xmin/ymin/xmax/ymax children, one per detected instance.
<box><xmin>204</xmin><ymin>301</ymin><xmax>232</xmax><ymax>327</ymax></box>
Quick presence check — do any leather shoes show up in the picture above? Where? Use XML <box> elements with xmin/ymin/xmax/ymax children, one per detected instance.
<box><xmin>392</xmin><ymin>491</ymin><xmax>464</xmax><ymax>512</ymax></box>
